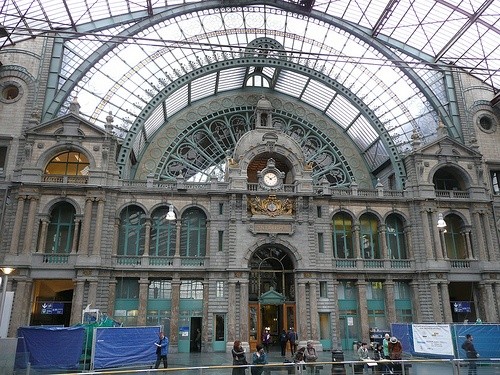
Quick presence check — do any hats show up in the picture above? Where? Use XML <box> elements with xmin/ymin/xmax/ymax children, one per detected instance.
<box><xmin>390</xmin><ymin>337</ymin><xmax>397</xmax><ymax>343</ymax></box>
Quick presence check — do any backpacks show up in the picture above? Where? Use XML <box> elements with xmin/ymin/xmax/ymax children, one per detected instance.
<box><xmin>281</xmin><ymin>332</ymin><xmax>287</xmax><ymax>341</ymax></box>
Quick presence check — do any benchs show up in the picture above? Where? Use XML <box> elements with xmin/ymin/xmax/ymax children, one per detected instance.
<box><xmin>349</xmin><ymin>364</ymin><xmax>412</xmax><ymax>375</ymax></box>
<box><xmin>297</xmin><ymin>365</ymin><xmax>323</xmax><ymax>375</ymax></box>
<box><xmin>264</xmin><ymin>357</ymin><xmax>295</xmax><ymax>375</ymax></box>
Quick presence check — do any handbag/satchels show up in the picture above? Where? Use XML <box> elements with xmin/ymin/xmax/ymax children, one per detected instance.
<box><xmin>295</xmin><ymin>340</ymin><xmax>299</xmax><ymax>345</ymax></box>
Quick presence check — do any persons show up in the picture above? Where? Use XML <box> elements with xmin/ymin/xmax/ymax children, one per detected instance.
<box><xmin>476</xmin><ymin>318</ymin><xmax>482</xmax><ymax>324</ymax></box>
<box><xmin>153</xmin><ymin>332</ymin><xmax>169</xmax><ymax>369</ymax></box>
<box><xmin>231</xmin><ymin>339</ymin><xmax>246</xmax><ymax>375</ymax></box>
<box><xmin>464</xmin><ymin>319</ymin><xmax>469</xmax><ymax>324</ymax></box>
<box><xmin>263</xmin><ymin>326</ymin><xmax>319</xmax><ymax>373</ymax></box>
<box><xmin>196</xmin><ymin>328</ymin><xmax>201</xmax><ymax>353</ymax></box>
<box><xmin>251</xmin><ymin>344</ymin><xmax>268</xmax><ymax>375</ymax></box>
<box><xmin>461</xmin><ymin>334</ymin><xmax>480</xmax><ymax>375</ymax></box>
<box><xmin>358</xmin><ymin>328</ymin><xmax>402</xmax><ymax>375</ymax></box>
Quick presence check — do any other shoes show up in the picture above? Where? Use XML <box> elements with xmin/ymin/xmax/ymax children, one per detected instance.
<box><xmin>389</xmin><ymin>370</ymin><xmax>393</xmax><ymax>374</ymax></box>
<box><xmin>291</xmin><ymin>355</ymin><xmax>294</xmax><ymax>357</ymax></box>
<box><xmin>382</xmin><ymin>373</ymin><xmax>384</xmax><ymax>375</ymax></box>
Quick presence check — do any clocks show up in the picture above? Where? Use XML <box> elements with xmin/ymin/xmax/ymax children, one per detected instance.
<box><xmin>264</xmin><ymin>173</ymin><xmax>277</xmax><ymax>186</ymax></box>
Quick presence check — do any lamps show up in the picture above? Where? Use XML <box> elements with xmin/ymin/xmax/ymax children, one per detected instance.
<box><xmin>165</xmin><ymin>192</ymin><xmax>176</xmax><ymax>220</ymax></box>
<box><xmin>437</xmin><ymin>198</ymin><xmax>447</xmax><ymax>227</ymax></box>
